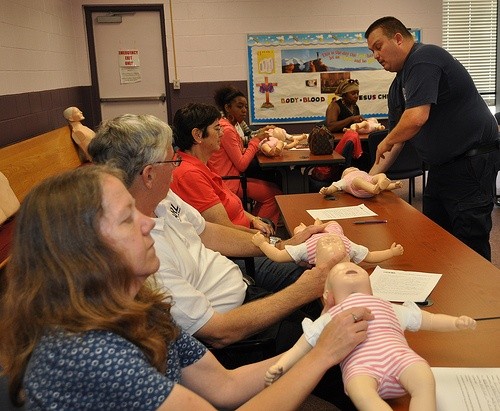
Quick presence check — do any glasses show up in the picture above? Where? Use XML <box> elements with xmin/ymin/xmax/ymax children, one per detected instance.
<box><xmin>140</xmin><ymin>153</ymin><xmax>182</xmax><ymax>175</ymax></box>
<box><xmin>348</xmin><ymin>79</ymin><xmax>359</xmax><ymax>85</ymax></box>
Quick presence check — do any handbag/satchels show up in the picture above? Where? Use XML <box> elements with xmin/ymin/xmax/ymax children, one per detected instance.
<box><xmin>308</xmin><ymin>125</ymin><xmax>335</xmax><ymax>155</ymax></box>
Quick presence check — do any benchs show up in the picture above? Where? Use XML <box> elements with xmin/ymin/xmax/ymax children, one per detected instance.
<box><xmin>0</xmin><ymin>124</ymin><xmax>91</xmax><ymax>293</ymax></box>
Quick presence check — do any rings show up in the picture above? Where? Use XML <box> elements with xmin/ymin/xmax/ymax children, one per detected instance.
<box><xmin>351</xmin><ymin>314</ymin><xmax>357</xmax><ymax>321</ymax></box>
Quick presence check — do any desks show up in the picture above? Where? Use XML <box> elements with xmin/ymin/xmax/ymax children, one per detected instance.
<box><xmin>255</xmin><ymin>133</ymin><xmax>345</xmax><ymax>195</ymax></box>
<box><xmin>390</xmin><ymin>320</ymin><xmax>500</xmax><ymax>411</ymax></box>
<box><xmin>275</xmin><ymin>189</ymin><xmax>500</xmax><ymax>320</ymax></box>
<box><xmin>332</xmin><ymin>132</ymin><xmax>370</xmax><ymax>141</ymax></box>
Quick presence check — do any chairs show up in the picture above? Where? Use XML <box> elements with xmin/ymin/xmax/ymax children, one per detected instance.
<box><xmin>367</xmin><ymin>130</ymin><xmax>425</xmax><ymax>205</ymax></box>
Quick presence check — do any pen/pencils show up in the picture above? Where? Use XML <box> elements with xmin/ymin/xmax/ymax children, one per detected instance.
<box><xmin>353</xmin><ymin>220</ymin><xmax>388</xmax><ymax>224</ymax></box>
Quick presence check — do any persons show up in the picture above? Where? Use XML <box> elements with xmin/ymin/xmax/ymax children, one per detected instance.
<box><xmin>63</xmin><ymin>106</ymin><xmax>97</xmax><ymax>161</ymax></box>
<box><xmin>326</xmin><ymin>78</ymin><xmax>368</xmax><ymax>132</ymax></box>
<box><xmin>253</xmin><ymin>125</ymin><xmax>307</xmax><ymax>157</ymax></box>
<box><xmin>251</xmin><ymin>216</ymin><xmax>405</xmax><ymax>266</ymax></box>
<box><xmin>364</xmin><ymin>16</ymin><xmax>500</xmax><ymax>263</ymax></box>
<box><xmin>0</xmin><ymin>164</ymin><xmax>376</xmax><ymax>410</ymax></box>
<box><xmin>87</xmin><ymin>114</ymin><xmax>348</xmax><ymax>401</ymax></box>
<box><xmin>169</xmin><ymin>104</ymin><xmax>313</xmax><ymax>293</ymax></box>
<box><xmin>264</xmin><ymin>262</ymin><xmax>477</xmax><ymax>411</ymax></box>
<box><xmin>207</xmin><ymin>86</ymin><xmax>284</xmax><ymax>234</ymax></box>
<box><xmin>319</xmin><ymin>167</ymin><xmax>404</xmax><ymax>198</ymax></box>
<box><xmin>343</xmin><ymin>118</ymin><xmax>385</xmax><ymax>133</ymax></box>
